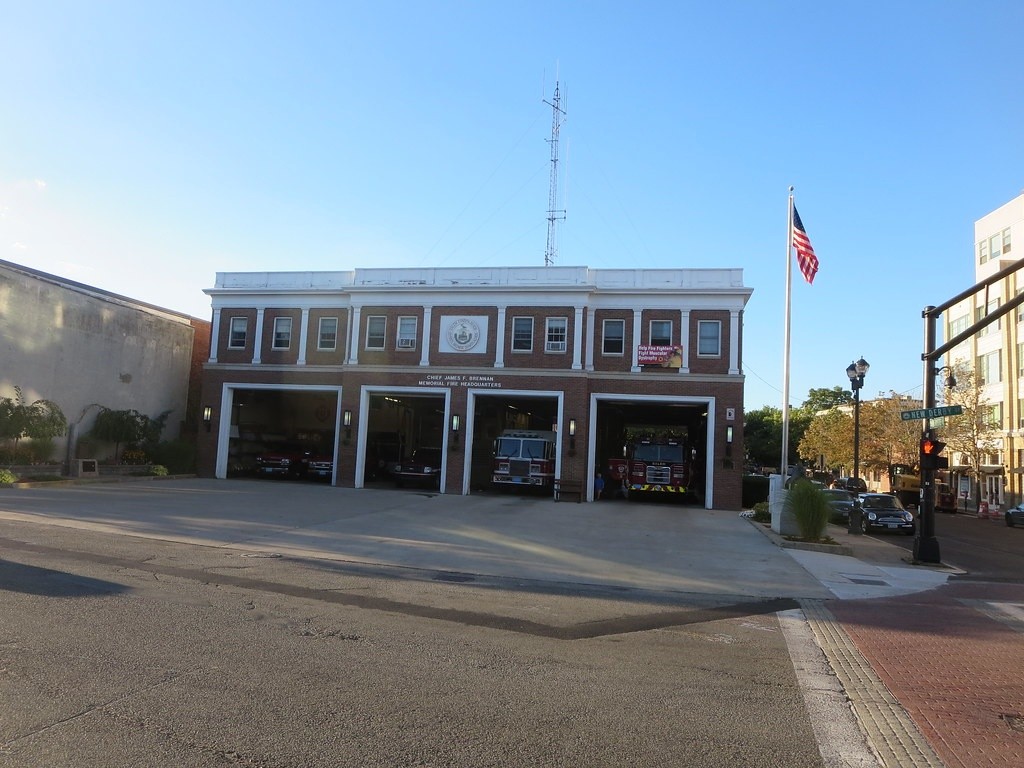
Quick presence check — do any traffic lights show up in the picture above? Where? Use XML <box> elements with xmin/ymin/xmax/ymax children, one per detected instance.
<box><xmin>933</xmin><ymin>439</ymin><xmax>949</xmax><ymax>471</ymax></box>
<box><xmin>920</xmin><ymin>436</ymin><xmax>939</xmax><ymax>470</ymax></box>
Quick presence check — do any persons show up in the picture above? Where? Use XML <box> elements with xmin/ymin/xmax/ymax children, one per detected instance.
<box><xmin>595</xmin><ymin>473</ymin><xmax>604</xmax><ymax>500</ymax></box>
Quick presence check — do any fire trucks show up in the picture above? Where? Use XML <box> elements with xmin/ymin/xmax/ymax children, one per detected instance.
<box><xmin>607</xmin><ymin>426</ymin><xmax>697</xmax><ymax>494</ymax></box>
<box><xmin>489</xmin><ymin>428</ymin><xmax>557</xmax><ymax>488</ymax></box>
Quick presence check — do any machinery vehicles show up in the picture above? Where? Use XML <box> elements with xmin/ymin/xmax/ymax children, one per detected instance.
<box><xmin>888</xmin><ymin>463</ymin><xmax>958</xmax><ymax>514</ymax></box>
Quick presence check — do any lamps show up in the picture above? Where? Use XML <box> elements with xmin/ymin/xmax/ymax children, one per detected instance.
<box><xmin>568</xmin><ymin>417</ymin><xmax>578</xmax><ymax>455</ymax></box>
<box><xmin>452</xmin><ymin>414</ymin><xmax>461</xmax><ymax>451</ymax></box>
<box><xmin>203</xmin><ymin>406</ymin><xmax>213</xmax><ymax>434</ymax></box>
<box><xmin>724</xmin><ymin>423</ymin><xmax>735</xmax><ymax>470</ymax></box>
<box><xmin>343</xmin><ymin>409</ymin><xmax>353</xmax><ymax>446</ymax></box>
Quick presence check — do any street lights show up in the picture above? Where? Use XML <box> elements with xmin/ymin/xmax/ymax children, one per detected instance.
<box><xmin>845</xmin><ymin>355</ymin><xmax>872</xmax><ymax>535</ymax></box>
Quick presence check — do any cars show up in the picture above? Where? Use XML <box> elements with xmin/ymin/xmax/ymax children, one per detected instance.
<box><xmin>847</xmin><ymin>492</ymin><xmax>916</xmax><ymax>537</ymax></box>
<box><xmin>257</xmin><ymin>448</ymin><xmax>334</xmax><ymax>478</ymax></box>
<box><xmin>817</xmin><ymin>488</ymin><xmax>857</xmax><ymax>523</ymax></box>
<box><xmin>393</xmin><ymin>446</ymin><xmax>442</xmax><ymax>486</ymax></box>
<box><xmin>1004</xmin><ymin>502</ymin><xmax>1024</xmax><ymax>526</ymax></box>
<box><xmin>746</xmin><ymin>464</ymin><xmax>868</xmax><ymax>493</ymax></box>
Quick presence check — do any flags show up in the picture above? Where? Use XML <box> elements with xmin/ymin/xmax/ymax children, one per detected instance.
<box><xmin>793</xmin><ymin>205</ymin><xmax>820</xmax><ymax>284</ymax></box>
